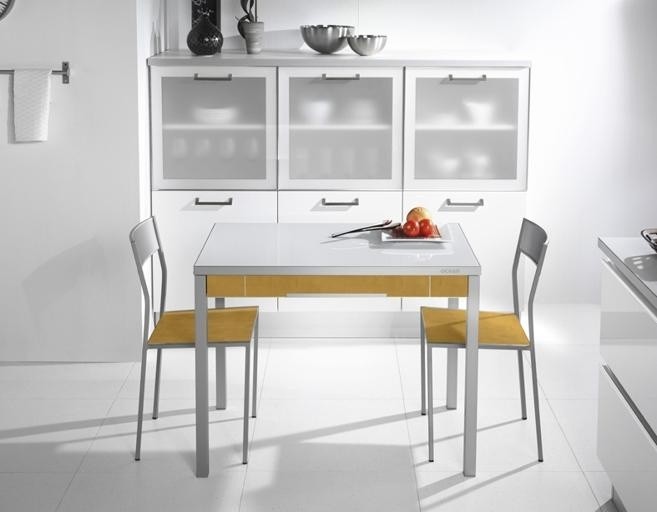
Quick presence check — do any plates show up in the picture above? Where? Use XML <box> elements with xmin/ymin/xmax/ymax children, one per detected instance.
<box><xmin>381</xmin><ymin>221</ymin><xmax>455</xmax><ymax>243</ymax></box>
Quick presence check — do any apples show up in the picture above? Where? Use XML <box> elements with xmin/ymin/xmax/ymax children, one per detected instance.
<box><xmin>406</xmin><ymin>206</ymin><xmax>433</xmax><ymax>223</ymax></box>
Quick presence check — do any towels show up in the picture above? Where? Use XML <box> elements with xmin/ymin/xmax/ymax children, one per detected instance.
<box><xmin>10</xmin><ymin>67</ymin><xmax>51</xmax><ymax>144</ymax></box>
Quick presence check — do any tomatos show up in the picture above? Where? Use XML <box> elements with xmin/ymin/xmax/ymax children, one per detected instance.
<box><xmin>403</xmin><ymin>220</ymin><xmax>419</xmax><ymax>236</ymax></box>
<box><xmin>418</xmin><ymin>219</ymin><xmax>433</xmax><ymax>236</ymax></box>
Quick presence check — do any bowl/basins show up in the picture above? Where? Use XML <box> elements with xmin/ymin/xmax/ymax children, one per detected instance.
<box><xmin>300</xmin><ymin>24</ymin><xmax>356</xmax><ymax>54</ymax></box>
<box><xmin>347</xmin><ymin>34</ymin><xmax>387</xmax><ymax>55</ymax></box>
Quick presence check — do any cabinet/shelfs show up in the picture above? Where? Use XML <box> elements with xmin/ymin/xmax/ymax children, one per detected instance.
<box><xmin>405</xmin><ymin>65</ymin><xmax>529</xmax><ymax>193</ymax></box>
<box><xmin>595</xmin><ymin>235</ymin><xmax>656</xmax><ymax>511</ymax></box>
<box><xmin>152</xmin><ymin>189</ymin><xmax>277</xmax><ymax>317</ymax></box>
<box><xmin>277</xmin><ymin>68</ymin><xmax>399</xmax><ymax>194</ymax></box>
<box><xmin>398</xmin><ymin>193</ymin><xmax>524</xmax><ymax>316</ymax></box>
<box><xmin>147</xmin><ymin>71</ymin><xmax>277</xmax><ymax>195</ymax></box>
<box><xmin>272</xmin><ymin>192</ymin><xmax>403</xmax><ymax>316</ymax></box>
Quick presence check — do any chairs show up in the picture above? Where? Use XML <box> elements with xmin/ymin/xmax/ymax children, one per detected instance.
<box><xmin>420</xmin><ymin>215</ymin><xmax>550</xmax><ymax>463</ymax></box>
<box><xmin>129</xmin><ymin>214</ymin><xmax>260</xmax><ymax>465</ymax></box>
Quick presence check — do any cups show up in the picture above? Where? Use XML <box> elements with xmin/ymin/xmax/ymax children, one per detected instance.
<box><xmin>242</xmin><ymin>22</ymin><xmax>264</xmax><ymax>54</ymax></box>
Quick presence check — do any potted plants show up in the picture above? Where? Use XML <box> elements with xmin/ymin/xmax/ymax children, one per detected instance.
<box><xmin>234</xmin><ymin>0</ymin><xmax>265</xmax><ymax>55</ymax></box>
